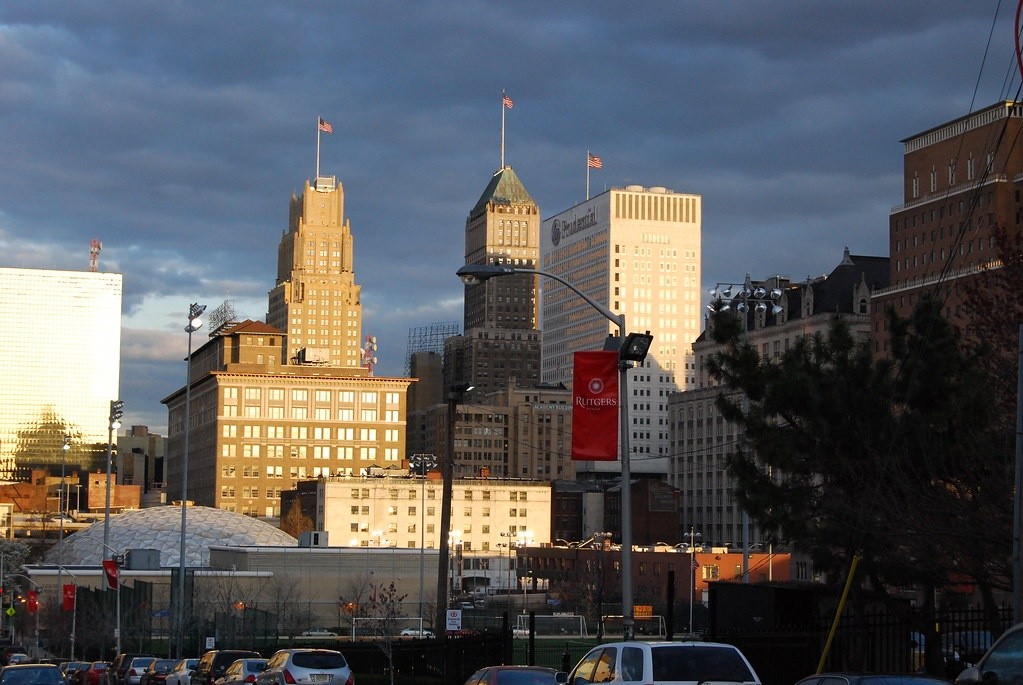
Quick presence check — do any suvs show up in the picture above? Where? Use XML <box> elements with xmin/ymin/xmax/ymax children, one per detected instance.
<box><xmin>254</xmin><ymin>647</ymin><xmax>355</xmax><ymax>685</ymax></box>
<box><xmin>554</xmin><ymin>640</ymin><xmax>762</xmax><ymax>685</ymax></box>
<box><xmin>955</xmin><ymin>622</ymin><xmax>1023</xmax><ymax>685</ymax></box>
<box><xmin>188</xmin><ymin>650</ymin><xmax>260</xmax><ymax>685</ymax></box>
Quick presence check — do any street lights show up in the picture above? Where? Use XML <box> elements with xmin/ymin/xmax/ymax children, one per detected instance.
<box><xmin>98</xmin><ymin>400</ymin><xmax>124</xmax><ymax>659</ymax></box>
<box><xmin>56</xmin><ymin>434</ymin><xmax>72</xmax><ymax>656</ymax></box>
<box><xmin>706</xmin><ymin>271</ymin><xmax>783</xmax><ymax>582</ymax></box>
<box><xmin>38</xmin><ymin>562</ymin><xmax>77</xmax><ymax>662</ymax></box>
<box><xmin>593</xmin><ymin>531</ymin><xmax>612</xmax><ymax>645</ymax></box>
<box><xmin>683</xmin><ymin>526</ymin><xmax>702</xmax><ymax>636</ymax></box>
<box><xmin>497</xmin><ymin>532</ymin><xmax>518</xmax><ymax>627</ymax></box>
<box><xmin>408</xmin><ymin>453</ymin><xmax>437</xmax><ymax>624</ymax></box>
<box><xmin>76</xmin><ymin>534</ymin><xmax>121</xmax><ymax>656</ymax></box>
<box><xmin>456</xmin><ymin>262</ymin><xmax>636</xmax><ymax>641</ymax></box>
<box><xmin>175</xmin><ymin>301</ymin><xmax>208</xmax><ymax>654</ymax></box>
<box><xmin>435</xmin><ymin>382</ymin><xmax>474</xmax><ymax>637</ymax></box>
<box><xmin>3</xmin><ymin>573</ymin><xmax>39</xmax><ymax>663</ymax></box>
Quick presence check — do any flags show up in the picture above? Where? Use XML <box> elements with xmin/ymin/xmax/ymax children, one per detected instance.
<box><xmin>504</xmin><ymin>91</ymin><xmax>513</xmax><ymax>109</ymax></box>
<box><xmin>320</xmin><ymin>118</ymin><xmax>332</xmax><ymax>134</ymax></box>
<box><xmin>589</xmin><ymin>153</ymin><xmax>602</xmax><ymax>169</ymax></box>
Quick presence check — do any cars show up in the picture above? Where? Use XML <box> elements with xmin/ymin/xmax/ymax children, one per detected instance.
<box><xmin>552</xmin><ymin>540</ymin><xmax>769</xmax><ymax>555</ymax></box>
<box><xmin>464</xmin><ymin>665</ymin><xmax>565</xmax><ymax>685</ymax></box>
<box><xmin>0</xmin><ymin>663</ymin><xmax>69</xmax><ymax>685</ymax></box>
<box><xmin>794</xmin><ymin>672</ymin><xmax>954</xmax><ymax>685</ymax></box>
<box><xmin>445</xmin><ymin>627</ymin><xmax>479</xmax><ymax>638</ymax></box>
<box><xmin>213</xmin><ymin>658</ymin><xmax>270</xmax><ymax>685</ymax></box>
<box><xmin>0</xmin><ymin>627</ymin><xmax>158</xmax><ymax>685</ymax></box>
<box><xmin>509</xmin><ymin>625</ymin><xmax>536</xmax><ymax>636</ymax></box>
<box><xmin>163</xmin><ymin>659</ymin><xmax>200</xmax><ymax>685</ymax></box>
<box><xmin>401</xmin><ymin>626</ymin><xmax>435</xmax><ymax>636</ymax></box>
<box><xmin>302</xmin><ymin>627</ymin><xmax>338</xmax><ymax>637</ymax></box>
<box><xmin>140</xmin><ymin>658</ymin><xmax>183</xmax><ymax>685</ymax></box>
